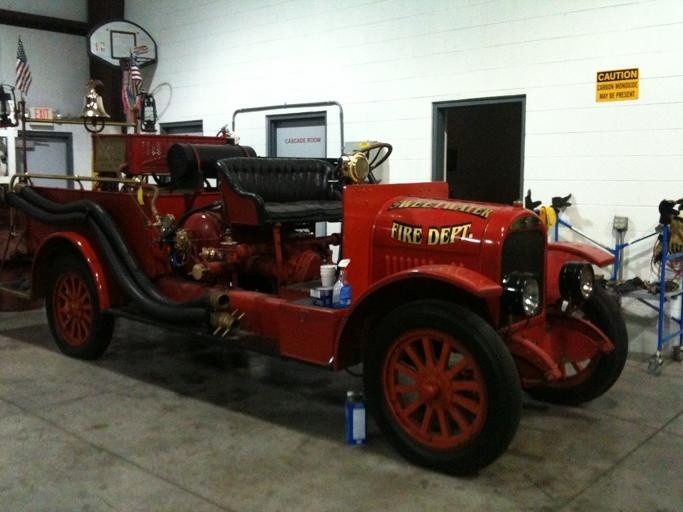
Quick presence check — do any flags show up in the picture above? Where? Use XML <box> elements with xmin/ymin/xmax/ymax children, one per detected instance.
<box><xmin>14</xmin><ymin>36</ymin><xmax>32</xmax><ymax>96</ymax></box>
<box><xmin>126</xmin><ymin>54</ymin><xmax>143</xmax><ymax>111</ymax></box>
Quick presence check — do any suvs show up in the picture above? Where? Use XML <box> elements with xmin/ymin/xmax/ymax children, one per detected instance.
<box><xmin>0</xmin><ymin>92</ymin><xmax>629</xmax><ymax>475</ymax></box>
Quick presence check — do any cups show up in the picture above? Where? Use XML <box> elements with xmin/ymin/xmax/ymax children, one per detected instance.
<box><xmin>319</xmin><ymin>264</ymin><xmax>336</xmax><ymax>289</ymax></box>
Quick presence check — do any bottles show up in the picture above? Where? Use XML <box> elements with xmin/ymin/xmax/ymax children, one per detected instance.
<box><xmin>344</xmin><ymin>389</ymin><xmax>365</xmax><ymax>446</ymax></box>
<box><xmin>331</xmin><ymin>268</ymin><xmax>352</xmax><ymax>308</ymax></box>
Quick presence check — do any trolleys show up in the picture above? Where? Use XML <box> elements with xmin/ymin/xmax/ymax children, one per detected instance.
<box><xmin>522</xmin><ymin>188</ymin><xmax>683</xmax><ymax>375</ymax></box>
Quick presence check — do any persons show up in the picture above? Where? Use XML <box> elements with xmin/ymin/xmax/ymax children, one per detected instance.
<box><xmin>80</xmin><ymin>79</ymin><xmax>110</xmax><ymax>118</ymax></box>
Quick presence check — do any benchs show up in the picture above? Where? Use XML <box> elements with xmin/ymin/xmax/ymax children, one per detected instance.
<box><xmin>214</xmin><ymin>155</ymin><xmax>343</xmax><ymax>296</ymax></box>
